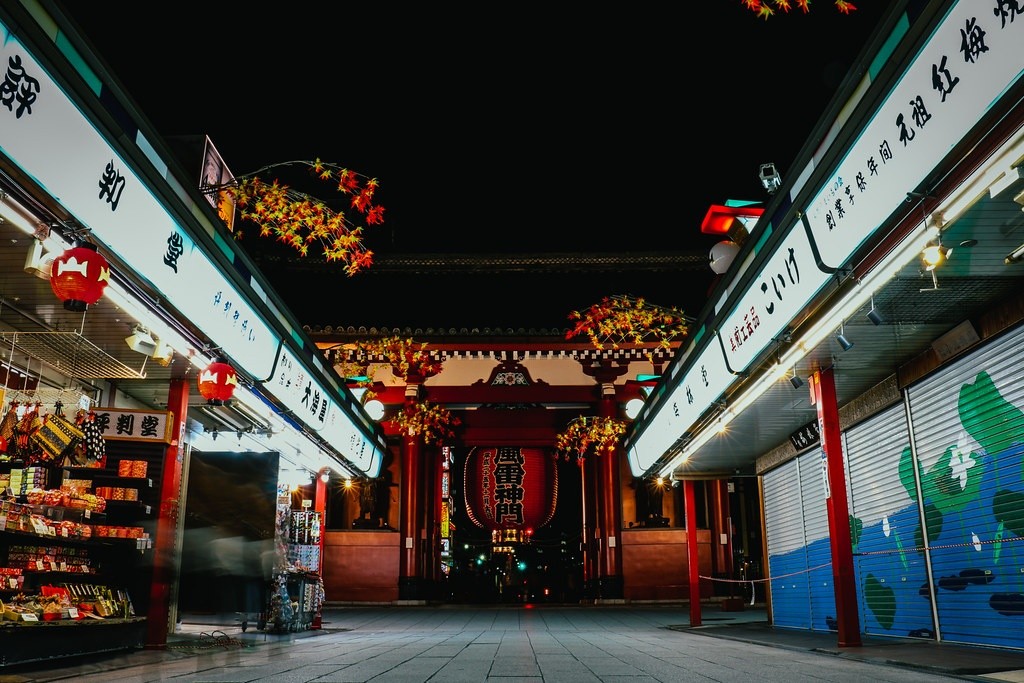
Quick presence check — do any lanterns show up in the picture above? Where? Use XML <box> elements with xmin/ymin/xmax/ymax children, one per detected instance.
<box><xmin>50</xmin><ymin>241</ymin><xmax>109</xmax><ymax>312</ymax></box>
<box><xmin>198</xmin><ymin>357</ymin><xmax>236</xmax><ymax>406</ymax></box>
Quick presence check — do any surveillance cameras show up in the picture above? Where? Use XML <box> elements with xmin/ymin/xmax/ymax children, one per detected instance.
<box><xmin>757</xmin><ymin>162</ymin><xmax>777</xmax><ymax>180</ymax></box>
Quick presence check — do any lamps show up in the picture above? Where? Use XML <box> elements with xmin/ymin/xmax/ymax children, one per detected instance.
<box><xmin>363</xmin><ymin>400</ymin><xmax>384</xmax><ymax>426</ymax></box>
<box><xmin>125</xmin><ymin>330</ymin><xmax>158</xmax><ymax>358</ymax></box>
<box><xmin>669</xmin><ymin>475</ymin><xmax>681</xmax><ymax>489</ymax></box>
<box><xmin>625</xmin><ymin>398</ymin><xmax>645</xmax><ymax>419</ymax></box>
<box><xmin>866</xmin><ymin>299</ymin><xmax>884</xmax><ymax>326</ymax></box>
<box><xmin>789</xmin><ymin>365</ymin><xmax>804</xmax><ymax>389</ymax></box>
<box><xmin>835</xmin><ymin>325</ymin><xmax>855</xmax><ymax>352</ymax></box>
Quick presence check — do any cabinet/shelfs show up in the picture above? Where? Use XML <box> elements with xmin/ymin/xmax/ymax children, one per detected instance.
<box><xmin>0</xmin><ymin>528</ymin><xmax>148</xmax><ymax>676</ymax></box>
<box><xmin>49</xmin><ymin>438</ymin><xmax>171</xmax><ymax>617</ymax></box>
